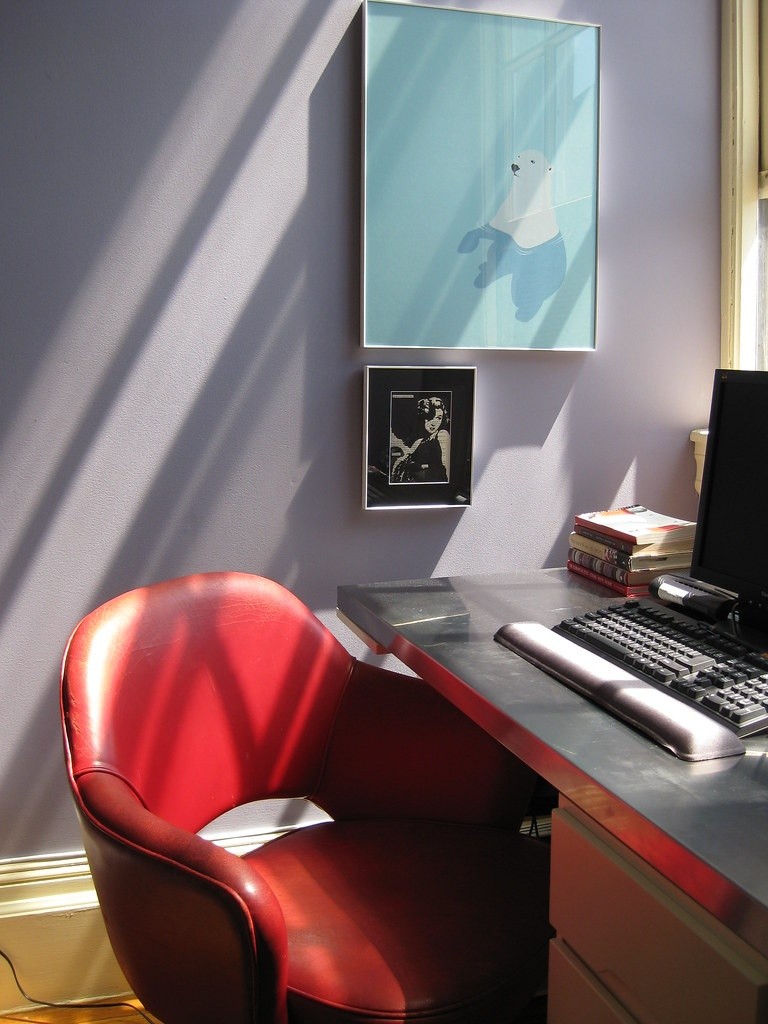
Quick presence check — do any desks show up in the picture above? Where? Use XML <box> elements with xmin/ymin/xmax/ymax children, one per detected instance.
<box><xmin>337</xmin><ymin>567</ymin><xmax>768</xmax><ymax>1024</ymax></box>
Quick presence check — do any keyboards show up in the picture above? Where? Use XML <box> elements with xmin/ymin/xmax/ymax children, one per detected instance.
<box><xmin>551</xmin><ymin>599</ymin><xmax>768</xmax><ymax>740</ymax></box>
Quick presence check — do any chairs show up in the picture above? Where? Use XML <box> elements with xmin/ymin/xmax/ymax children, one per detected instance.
<box><xmin>60</xmin><ymin>570</ymin><xmax>550</xmax><ymax>1024</ymax></box>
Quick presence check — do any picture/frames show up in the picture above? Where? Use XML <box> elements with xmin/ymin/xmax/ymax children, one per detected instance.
<box><xmin>361</xmin><ymin>365</ymin><xmax>477</xmax><ymax>510</ymax></box>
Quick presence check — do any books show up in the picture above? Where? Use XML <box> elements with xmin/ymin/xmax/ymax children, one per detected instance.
<box><xmin>567</xmin><ymin>523</ymin><xmax>695</xmax><ymax>597</ymax></box>
<box><xmin>574</xmin><ymin>504</ymin><xmax>697</xmax><ymax>545</ymax></box>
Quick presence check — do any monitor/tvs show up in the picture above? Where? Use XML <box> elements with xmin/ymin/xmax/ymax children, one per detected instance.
<box><xmin>688</xmin><ymin>368</ymin><xmax>768</xmax><ymax>655</ymax></box>
